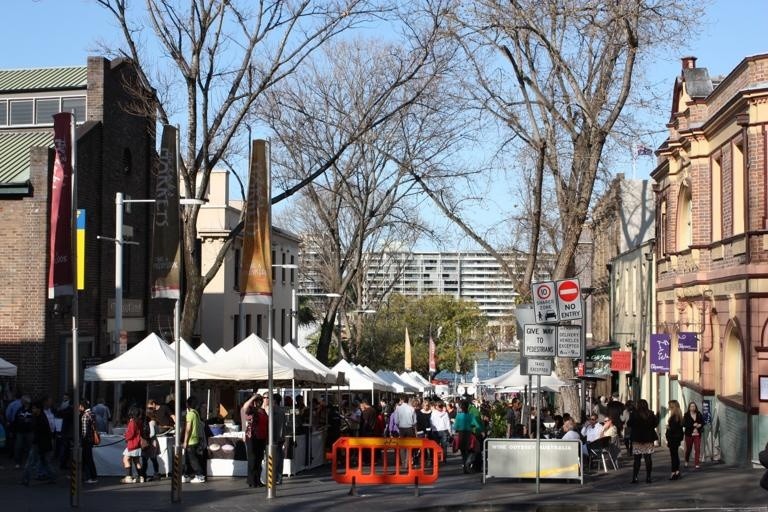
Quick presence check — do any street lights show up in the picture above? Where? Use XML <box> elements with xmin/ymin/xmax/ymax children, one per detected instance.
<box><xmin>287</xmin><ymin>287</ymin><xmax>338</xmax><ymax>349</ymax></box>
<box><xmin>578</xmin><ymin>285</ymin><xmax>597</xmax><ymax>417</ymax></box>
<box><xmin>265</xmin><ymin>263</ymin><xmax>298</xmax><ymax>503</ymax></box>
<box><xmin>346</xmin><ymin>309</ymin><xmax>376</xmax><ymax>316</ymax></box>
<box><xmin>95</xmin><ymin>191</ymin><xmax>207</xmax><ymax>428</ymax></box>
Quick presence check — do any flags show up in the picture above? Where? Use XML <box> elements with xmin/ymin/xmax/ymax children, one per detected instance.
<box><xmin>429</xmin><ymin>335</ymin><xmax>436</xmax><ymax>372</ymax></box>
<box><xmin>49</xmin><ymin>112</ymin><xmax>77</xmax><ymax>299</ymax></box>
<box><xmin>148</xmin><ymin>124</ymin><xmax>181</xmax><ymax>300</ymax></box>
<box><xmin>404</xmin><ymin>325</ymin><xmax>412</xmax><ymax>371</ymax></box>
<box><xmin>239</xmin><ymin>140</ymin><xmax>273</xmax><ymax>304</ymax></box>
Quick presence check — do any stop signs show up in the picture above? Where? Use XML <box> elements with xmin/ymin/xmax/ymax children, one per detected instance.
<box><xmin>556</xmin><ymin>278</ymin><xmax>582</xmax><ymax>322</ymax></box>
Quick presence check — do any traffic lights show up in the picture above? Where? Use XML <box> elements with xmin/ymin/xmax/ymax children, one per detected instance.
<box><xmin>487</xmin><ymin>344</ymin><xmax>498</xmax><ymax>364</ymax></box>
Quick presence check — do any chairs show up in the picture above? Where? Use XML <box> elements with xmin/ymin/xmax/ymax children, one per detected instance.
<box><xmin>587</xmin><ymin>434</ymin><xmax>623</xmax><ymax>474</ymax></box>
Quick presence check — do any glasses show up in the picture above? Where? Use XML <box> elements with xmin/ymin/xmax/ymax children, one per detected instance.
<box><xmin>262</xmin><ymin>397</ymin><xmax>268</xmax><ymax>400</ymax></box>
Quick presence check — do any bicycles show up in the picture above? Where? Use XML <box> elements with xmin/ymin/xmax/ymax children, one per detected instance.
<box><xmin>22</xmin><ymin>445</ymin><xmax>58</xmax><ymax>486</ymax></box>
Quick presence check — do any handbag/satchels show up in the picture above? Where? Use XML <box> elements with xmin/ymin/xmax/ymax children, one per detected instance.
<box><xmin>86</xmin><ymin>411</ymin><xmax>100</xmax><ymax>445</ymax></box>
<box><xmin>193</xmin><ymin>412</ymin><xmax>208</xmax><ymax>450</ymax></box>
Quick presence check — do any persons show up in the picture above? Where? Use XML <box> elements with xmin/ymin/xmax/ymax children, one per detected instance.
<box><xmin>2</xmin><ymin>392</ymin><xmax>111</xmax><ymax>484</ymax></box>
<box><xmin>114</xmin><ymin>392</ymin><xmax>635</xmax><ymax>487</ymax></box>
<box><xmin>683</xmin><ymin>401</ymin><xmax>705</xmax><ymax>468</ymax></box>
<box><xmin>666</xmin><ymin>400</ymin><xmax>683</xmax><ymax>480</ymax></box>
<box><xmin>759</xmin><ymin>442</ymin><xmax>768</xmax><ymax>490</ymax></box>
<box><xmin>626</xmin><ymin>399</ymin><xmax>659</xmax><ymax>484</ymax></box>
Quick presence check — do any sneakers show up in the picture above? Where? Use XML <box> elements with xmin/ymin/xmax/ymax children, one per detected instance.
<box><xmin>84</xmin><ymin>478</ymin><xmax>98</xmax><ymax>484</ymax></box>
<box><xmin>119</xmin><ymin>475</ymin><xmax>133</xmax><ymax>483</ymax></box>
<box><xmin>190</xmin><ymin>475</ymin><xmax>206</xmax><ymax>483</ymax></box>
<box><xmin>133</xmin><ymin>476</ymin><xmax>145</xmax><ymax>482</ymax></box>
<box><xmin>182</xmin><ymin>475</ymin><xmax>190</xmax><ymax>483</ymax></box>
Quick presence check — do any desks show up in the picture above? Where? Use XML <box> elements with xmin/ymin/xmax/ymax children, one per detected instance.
<box><xmin>204</xmin><ymin>430</ymin><xmax>249</xmax><ymax>477</ymax></box>
<box><xmin>90</xmin><ymin>434</ymin><xmax>174</xmax><ymax>480</ymax></box>
<box><xmin>278</xmin><ymin>428</ymin><xmax>325</xmax><ymax>479</ymax></box>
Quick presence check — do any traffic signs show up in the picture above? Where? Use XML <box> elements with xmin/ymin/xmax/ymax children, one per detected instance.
<box><xmin>555</xmin><ymin>324</ymin><xmax>583</xmax><ymax>359</ymax></box>
<box><xmin>531</xmin><ymin>280</ymin><xmax>558</xmax><ymax>326</ymax></box>
<box><xmin>525</xmin><ymin>358</ymin><xmax>553</xmax><ymax>376</ymax></box>
<box><xmin>522</xmin><ymin>322</ymin><xmax>556</xmax><ymax>357</ymax></box>
